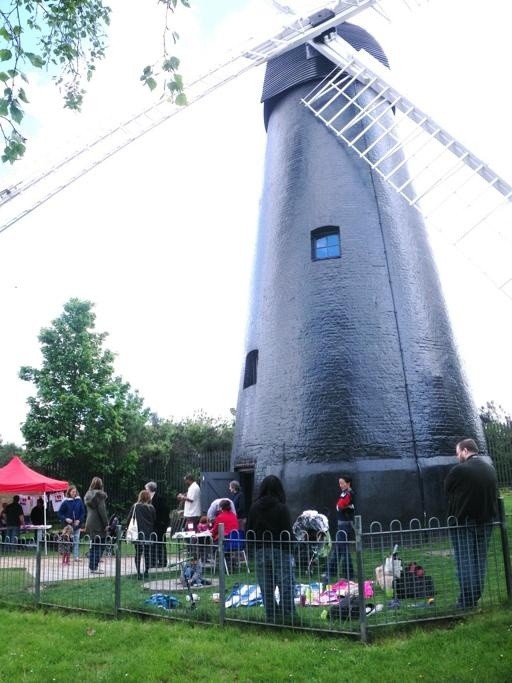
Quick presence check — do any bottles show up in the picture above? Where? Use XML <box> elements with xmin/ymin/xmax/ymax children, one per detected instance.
<box><xmin>183</xmin><ymin>592</ymin><xmax>223</xmax><ymax>603</ymax></box>
<box><xmin>390</xmin><ymin>544</ymin><xmax>400</xmax><ymax>560</ymax></box>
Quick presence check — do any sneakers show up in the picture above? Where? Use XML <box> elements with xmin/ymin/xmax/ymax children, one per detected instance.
<box><xmin>448</xmin><ymin>601</ymin><xmax>478</xmax><ymax>611</ymax></box>
<box><xmin>90</xmin><ymin>567</ymin><xmax>105</xmax><ymax>573</ymax></box>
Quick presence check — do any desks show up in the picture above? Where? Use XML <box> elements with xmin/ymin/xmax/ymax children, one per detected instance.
<box><xmin>1</xmin><ymin>524</ymin><xmax>53</xmax><ymax>550</ymax></box>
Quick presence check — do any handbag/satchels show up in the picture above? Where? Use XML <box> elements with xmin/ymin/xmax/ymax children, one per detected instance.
<box><xmin>328</xmin><ymin>556</ymin><xmax>437</xmax><ymax>620</ymax></box>
<box><xmin>126</xmin><ymin>517</ymin><xmax>139</xmax><ymax>543</ymax></box>
<box><xmin>145</xmin><ymin>593</ymin><xmax>180</xmax><ymax>609</ymax></box>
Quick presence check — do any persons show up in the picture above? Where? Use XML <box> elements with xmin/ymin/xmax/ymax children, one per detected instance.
<box><xmin>4</xmin><ymin>494</ymin><xmax>29</xmax><ymax>543</ymax></box>
<box><xmin>443</xmin><ymin>437</ymin><xmax>500</xmax><ymax>609</ymax></box>
<box><xmin>125</xmin><ymin>489</ymin><xmax>156</xmax><ymax>582</ymax></box>
<box><xmin>31</xmin><ymin>498</ymin><xmax>45</xmax><ymax>525</ymax></box>
<box><xmin>59</xmin><ymin>484</ymin><xmax>85</xmax><ymax>563</ymax></box>
<box><xmin>143</xmin><ymin>481</ymin><xmax>171</xmax><ymax>568</ymax></box>
<box><xmin>209</xmin><ymin>501</ymin><xmax>240</xmax><ymax>574</ymax></box>
<box><xmin>180</xmin><ymin>554</ymin><xmax>213</xmax><ymax>588</ymax></box>
<box><xmin>59</xmin><ymin>524</ymin><xmax>76</xmax><ymax>564</ymax></box>
<box><xmin>83</xmin><ymin>476</ymin><xmax>111</xmax><ymax>574</ymax></box>
<box><xmin>229</xmin><ymin>479</ymin><xmax>249</xmax><ymax>545</ymax></box>
<box><xmin>248</xmin><ymin>474</ymin><xmax>297</xmax><ymax>620</ymax></box>
<box><xmin>176</xmin><ymin>472</ymin><xmax>200</xmax><ymax>558</ymax></box>
<box><xmin>326</xmin><ymin>473</ymin><xmax>356</xmax><ymax>581</ymax></box>
<box><xmin>0</xmin><ymin>502</ymin><xmax>9</xmax><ymax>541</ymax></box>
<box><xmin>197</xmin><ymin>515</ymin><xmax>212</xmax><ymax>562</ymax></box>
<box><xmin>207</xmin><ymin>497</ymin><xmax>237</xmax><ymax>518</ymax></box>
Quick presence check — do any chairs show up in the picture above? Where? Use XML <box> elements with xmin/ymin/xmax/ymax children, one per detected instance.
<box><xmin>213</xmin><ymin>528</ymin><xmax>252</xmax><ymax>577</ymax></box>
